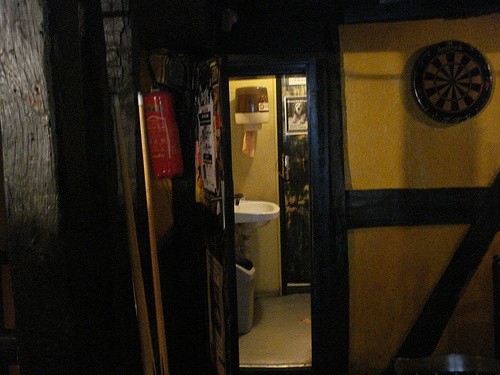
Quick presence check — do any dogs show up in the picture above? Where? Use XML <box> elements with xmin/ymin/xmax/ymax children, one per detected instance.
<box><xmin>292</xmin><ymin>102</ymin><xmax>307</xmax><ymax>124</ymax></box>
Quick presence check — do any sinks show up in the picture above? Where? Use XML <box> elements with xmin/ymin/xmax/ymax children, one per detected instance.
<box><xmin>235</xmin><ymin>200</ymin><xmax>280</xmax><ymax>223</ymax></box>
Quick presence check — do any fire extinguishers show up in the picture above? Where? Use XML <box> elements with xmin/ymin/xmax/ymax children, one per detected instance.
<box><xmin>141</xmin><ymin>78</ymin><xmax>182</xmax><ymax>183</ymax></box>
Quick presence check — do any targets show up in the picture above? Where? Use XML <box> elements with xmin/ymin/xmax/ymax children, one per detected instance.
<box><xmin>409</xmin><ymin>38</ymin><xmax>495</xmax><ymax>126</ymax></box>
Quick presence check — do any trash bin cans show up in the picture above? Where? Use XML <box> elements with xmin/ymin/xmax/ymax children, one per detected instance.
<box><xmin>234</xmin><ymin>257</ymin><xmax>257</xmax><ymax>332</ymax></box>
